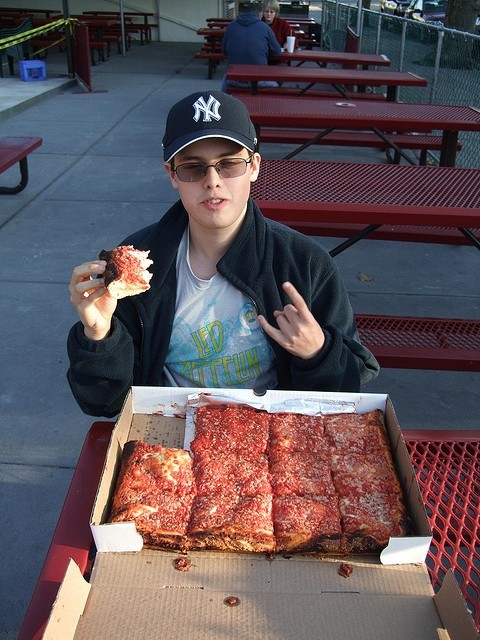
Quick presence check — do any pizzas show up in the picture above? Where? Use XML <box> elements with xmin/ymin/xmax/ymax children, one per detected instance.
<box><xmin>110</xmin><ymin>404</ymin><xmax>407</xmax><ymax>558</ymax></box>
<box><xmin>97</xmin><ymin>245</ymin><xmax>154</xmax><ymax>297</ymax></box>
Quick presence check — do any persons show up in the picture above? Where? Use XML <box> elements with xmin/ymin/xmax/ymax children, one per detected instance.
<box><xmin>67</xmin><ymin>90</ymin><xmax>381</xmax><ymax>418</ymax></box>
<box><xmin>220</xmin><ymin>1</ymin><xmax>284</xmax><ymax>94</ymax></box>
<box><xmin>261</xmin><ymin>1</ymin><xmax>297</xmax><ymax>50</ymax></box>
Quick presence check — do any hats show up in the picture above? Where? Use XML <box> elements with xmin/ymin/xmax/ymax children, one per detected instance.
<box><xmin>162</xmin><ymin>90</ymin><xmax>257</xmax><ymax>163</ymax></box>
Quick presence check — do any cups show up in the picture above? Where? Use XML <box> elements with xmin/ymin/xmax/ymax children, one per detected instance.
<box><xmin>286</xmin><ymin>36</ymin><xmax>296</xmax><ymax>53</ymax></box>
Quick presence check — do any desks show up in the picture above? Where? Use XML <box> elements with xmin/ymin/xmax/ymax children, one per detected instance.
<box><xmin>282</xmin><ymin>16</ymin><xmax>314</xmax><ymax>21</ymax></box>
<box><xmin>267</xmin><ymin>48</ymin><xmax>391</xmax><ymax>69</ymax></box>
<box><xmin>0</xmin><ymin>7</ymin><xmax>159</xmax><ymax>65</ymax></box>
<box><xmin>196</xmin><ymin>28</ymin><xmax>227</xmax><ymax>51</ymax></box>
<box><xmin>229</xmin><ymin>93</ymin><xmax>480</xmax><ymax>167</ymax></box>
<box><xmin>247</xmin><ymin>158</ymin><xmax>480</xmax><ymax>257</ymax></box>
<box><xmin>296</xmin><ymin>38</ymin><xmax>316</xmax><ymax>50</ymax></box>
<box><xmin>15</xmin><ymin>422</ymin><xmax>479</xmax><ymax>636</ymax></box>
<box><xmin>207</xmin><ymin>21</ymin><xmax>231</xmax><ymax>28</ymax></box>
<box><xmin>205</xmin><ymin>18</ymin><xmax>232</xmax><ymax>21</ymax></box>
<box><xmin>295</xmin><ymin>30</ymin><xmax>305</xmax><ymax>37</ymax></box>
<box><xmin>287</xmin><ymin>20</ymin><xmax>315</xmax><ymax>33</ymax></box>
<box><xmin>226</xmin><ymin>63</ymin><xmax>428</xmax><ymax>102</ymax></box>
<box><xmin>289</xmin><ymin>24</ymin><xmax>300</xmax><ymax>30</ymax></box>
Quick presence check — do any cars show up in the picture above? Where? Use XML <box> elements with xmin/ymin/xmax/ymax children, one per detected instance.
<box><xmin>403</xmin><ymin>0</ymin><xmax>445</xmax><ymax>24</ymax></box>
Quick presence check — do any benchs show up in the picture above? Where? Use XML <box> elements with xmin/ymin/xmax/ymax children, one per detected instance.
<box><xmin>271</xmin><ymin>220</ymin><xmax>480</xmax><ymax>250</ymax></box>
<box><xmin>2</xmin><ymin>13</ymin><xmax>157</xmax><ymax>64</ymax></box>
<box><xmin>226</xmin><ymin>87</ymin><xmax>385</xmax><ymax>99</ymax></box>
<box><xmin>204</xmin><ymin>42</ymin><xmax>222</xmax><ymax>46</ymax></box>
<box><xmin>201</xmin><ymin>46</ymin><xmax>224</xmax><ymax>53</ymax></box>
<box><xmin>1</xmin><ymin>135</ymin><xmax>43</xmax><ymax>195</ymax></box>
<box><xmin>353</xmin><ymin>316</ymin><xmax>479</xmax><ymax>374</ymax></box>
<box><xmin>192</xmin><ymin>51</ymin><xmax>228</xmax><ymax>79</ymax></box>
<box><xmin>258</xmin><ymin>128</ymin><xmax>462</xmax><ymax>166</ymax></box>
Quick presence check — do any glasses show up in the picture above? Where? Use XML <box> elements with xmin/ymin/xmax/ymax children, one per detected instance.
<box><xmin>170</xmin><ymin>153</ymin><xmax>253</xmax><ymax>183</ymax></box>
